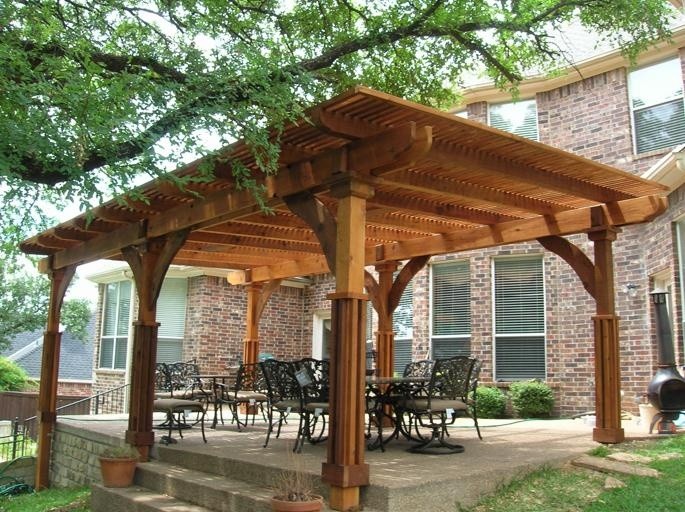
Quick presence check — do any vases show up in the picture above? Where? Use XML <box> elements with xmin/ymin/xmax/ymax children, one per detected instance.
<box><xmin>639</xmin><ymin>404</ymin><xmax>661</xmax><ymax>430</ymax></box>
<box><xmin>270</xmin><ymin>495</ymin><xmax>324</xmax><ymax>512</ymax></box>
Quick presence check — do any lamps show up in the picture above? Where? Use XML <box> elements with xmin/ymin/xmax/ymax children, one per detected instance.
<box><xmin>621</xmin><ymin>281</ymin><xmax>641</xmax><ymax>297</ymax></box>
<box><xmin>649</xmin><ymin>288</ymin><xmax>671</xmax><ymax>304</ymax></box>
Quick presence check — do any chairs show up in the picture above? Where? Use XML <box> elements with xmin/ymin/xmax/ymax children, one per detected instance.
<box><xmin>150</xmin><ymin>358</ymin><xmax>330</xmax><ymax>453</ymax></box>
<box><xmin>365</xmin><ymin>354</ymin><xmax>482</xmax><ymax>454</ymax></box>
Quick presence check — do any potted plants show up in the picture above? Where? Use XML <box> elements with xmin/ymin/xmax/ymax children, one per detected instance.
<box><xmin>98</xmin><ymin>444</ymin><xmax>138</xmax><ymax>489</ymax></box>
